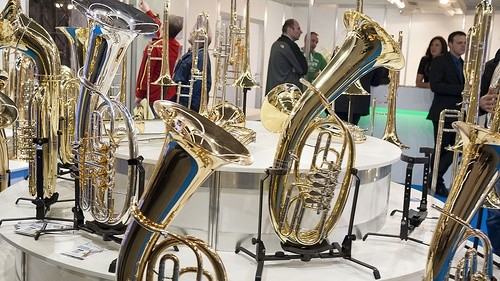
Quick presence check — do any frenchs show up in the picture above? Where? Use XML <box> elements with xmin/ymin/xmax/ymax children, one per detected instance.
<box><xmin>72</xmin><ymin>0</ymin><xmax>160</xmax><ymax>226</ymax></box>
<box><xmin>268</xmin><ymin>9</ymin><xmax>405</xmax><ymax>247</ymax></box>
<box><xmin>1</xmin><ymin>1</ymin><xmax>78</xmax><ymax>200</ymax></box>
<box><xmin>115</xmin><ymin>99</ymin><xmax>255</xmax><ymax>281</ymax></box>
<box><xmin>260</xmin><ymin>83</ymin><xmax>369</xmax><ymax>144</ymax></box>
<box><xmin>421</xmin><ymin>0</ymin><xmax>498</xmax><ymax>281</ymax></box>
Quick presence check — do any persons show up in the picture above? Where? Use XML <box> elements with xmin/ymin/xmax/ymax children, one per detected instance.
<box><xmin>171</xmin><ymin>26</ymin><xmax>212</xmax><ymax>115</ymax></box>
<box><xmin>265</xmin><ymin>18</ymin><xmax>308</xmax><ymax>109</ymax></box>
<box><xmin>135</xmin><ymin>0</ymin><xmax>183</xmax><ymax>119</ymax></box>
<box><xmin>476</xmin><ymin>48</ymin><xmax>500</xmax><ymax>258</ymax></box>
<box><xmin>413</xmin><ymin>36</ymin><xmax>448</xmax><ymax>88</ymax></box>
<box><xmin>302</xmin><ymin>29</ymin><xmax>390</xmax><ymax>128</ymax></box>
<box><xmin>426</xmin><ymin>31</ymin><xmax>466</xmax><ymax>199</ymax></box>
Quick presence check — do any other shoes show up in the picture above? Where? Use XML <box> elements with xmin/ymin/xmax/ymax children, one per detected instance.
<box><xmin>428</xmin><ymin>172</ymin><xmax>449</xmax><ymax>198</ymax></box>
